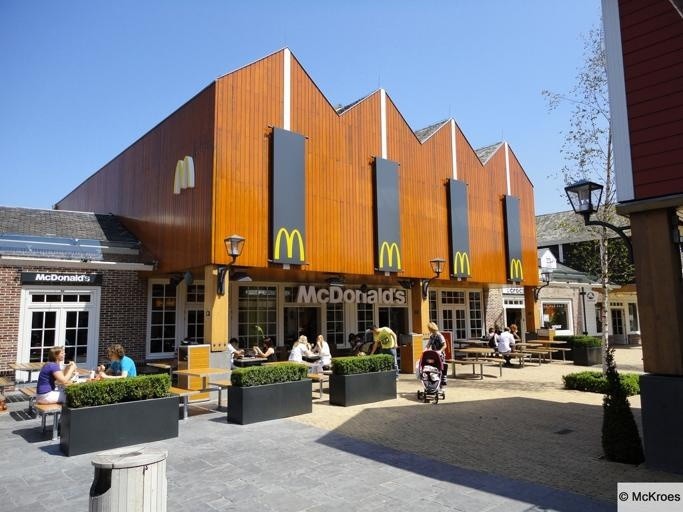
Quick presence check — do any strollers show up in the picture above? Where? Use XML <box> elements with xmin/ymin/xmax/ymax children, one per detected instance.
<box><xmin>414</xmin><ymin>347</ymin><xmax>448</xmax><ymax>405</ymax></box>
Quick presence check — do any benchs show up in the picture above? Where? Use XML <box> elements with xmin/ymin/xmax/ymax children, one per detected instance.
<box><xmin>0</xmin><ymin>329</ymin><xmax>577</xmax><ymax>440</ymax></box>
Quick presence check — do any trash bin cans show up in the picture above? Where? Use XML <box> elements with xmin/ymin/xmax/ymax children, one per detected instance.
<box><xmin>89</xmin><ymin>447</ymin><xmax>168</xmax><ymax>511</ymax></box>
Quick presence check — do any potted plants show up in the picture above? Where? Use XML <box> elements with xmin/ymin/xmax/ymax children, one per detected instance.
<box><xmin>570</xmin><ymin>333</ymin><xmax>605</xmax><ymax>367</ymax></box>
<box><xmin>55</xmin><ymin>370</ymin><xmax>179</xmax><ymax>456</ymax></box>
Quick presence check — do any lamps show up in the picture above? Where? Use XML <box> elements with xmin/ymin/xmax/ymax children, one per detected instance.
<box><xmin>420</xmin><ymin>256</ymin><xmax>449</xmax><ymax>301</ymax></box>
<box><xmin>216</xmin><ymin>234</ymin><xmax>249</xmax><ymax>298</ymax></box>
<box><xmin>565</xmin><ymin>176</ymin><xmax>632</xmax><ymax>263</ymax></box>
<box><xmin>536</xmin><ymin>267</ymin><xmax>553</xmax><ymax>302</ymax></box>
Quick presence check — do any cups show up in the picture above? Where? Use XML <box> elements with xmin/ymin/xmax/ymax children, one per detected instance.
<box><xmin>88</xmin><ymin>371</ymin><xmax>95</xmax><ymax>380</ymax></box>
<box><xmin>72</xmin><ymin>371</ymin><xmax>79</xmax><ymax>381</ymax></box>
<box><xmin>240</xmin><ymin>354</ymin><xmax>244</xmax><ymax>360</ymax></box>
<box><xmin>254</xmin><ymin>354</ymin><xmax>259</xmax><ymax>359</ymax></box>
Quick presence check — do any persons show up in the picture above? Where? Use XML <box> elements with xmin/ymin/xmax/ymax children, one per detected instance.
<box><xmin>310</xmin><ymin>333</ymin><xmax>331</xmax><ymax>374</ymax></box>
<box><xmin>287</xmin><ymin>335</ymin><xmax>312</xmax><ymax>363</ymax></box>
<box><xmin>252</xmin><ymin>337</ymin><xmax>276</xmax><ymax>362</ymax></box>
<box><xmin>93</xmin><ymin>344</ymin><xmax>137</xmax><ymax>380</ymax></box>
<box><xmin>34</xmin><ymin>345</ymin><xmax>76</xmax><ymax>433</ymax></box>
<box><xmin>485</xmin><ymin>322</ymin><xmax>520</xmax><ymax>366</ymax></box>
<box><xmin>251</xmin><ymin>323</ymin><xmax>266</xmax><ymax>345</ymax></box>
<box><xmin>425</xmin><ymin>320</ymin><xmax>447</xmax><ymax>368</ymax></box>
<box><xmin>227</xmin><ymin>337</ymin><xmax>244</xmax><ymax>370</ymax></box>
<box><xmin>366</xmin><ymin>323</ymin><xmax>401</xmax><ymax>382</ymax></box>
<box><xmin>347</xmin><ymin>331</ymin><xmax>364</xmax><ymax>356</ymax></box>
